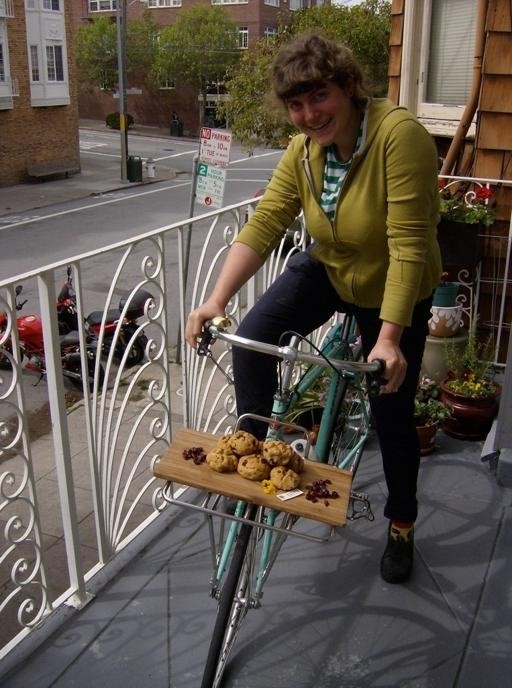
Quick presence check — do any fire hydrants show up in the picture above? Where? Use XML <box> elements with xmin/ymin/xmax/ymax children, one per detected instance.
<box><xmin>145</xmin><ymin>156</ymin><xmax>157</xmax><ymax>179</ymax></box>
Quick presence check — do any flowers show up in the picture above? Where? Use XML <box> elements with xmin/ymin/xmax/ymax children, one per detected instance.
<box><xmin>410</xmin><ymin>372</ymin><xmax>455</xmax><ymax>422</ymax></box>
<box><xmin>438</xmin><ymin>180</ymin><xmax>496</xmax><ymax>226</ymax></box>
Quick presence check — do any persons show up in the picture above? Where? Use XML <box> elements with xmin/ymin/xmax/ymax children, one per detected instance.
<box><xmin>184</xmin><ymin>30</ymin><xmax>445</xmax><ymax>584</ymax></box>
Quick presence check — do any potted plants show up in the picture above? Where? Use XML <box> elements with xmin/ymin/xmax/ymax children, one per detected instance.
<box><xmin>441</xmin><ymin>313</ymin><xmax>502</xmax><ymax>441</ymax></box>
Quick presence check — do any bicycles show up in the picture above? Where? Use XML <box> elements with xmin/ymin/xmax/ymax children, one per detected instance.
<box><xmin>197</xmin><ymin>302</ymin><xmax>386</xmax><ymax>688</ymax></box>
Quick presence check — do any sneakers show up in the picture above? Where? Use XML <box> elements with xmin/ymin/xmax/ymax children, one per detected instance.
<box><xmin>380</xmin><ymin>522</ymin><xmax>416</xmax><ymax>586</ymax></box>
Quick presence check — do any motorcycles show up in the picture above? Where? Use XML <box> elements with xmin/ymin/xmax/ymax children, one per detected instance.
<box><xmin>0</xmin><ymin>265</ymin><xmax>154</xmax><ymax>393</ymax></box>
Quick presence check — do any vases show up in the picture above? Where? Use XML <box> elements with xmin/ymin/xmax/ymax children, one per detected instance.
<box><xmin>333</xmin><ymin>398</ymin><xmax>370</xmax><ymax>449</ymax></box>
<box><xmin>436</xmin><ymin>217</ymin><xmax>482</xmax><ymax>268</ymax></box>
<box><xmin>431</xmin><ymin>282</ymin><xmax>459</xmax><ymax>308</ymax></box>
<box><xmin>414</xmin><ymin>416</ymin><xmax>439</xmax><ymax>453</ymax></box>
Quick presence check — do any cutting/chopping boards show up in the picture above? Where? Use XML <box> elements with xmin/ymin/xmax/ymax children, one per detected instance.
<box><xmin>153</xmin><ymin>430</ymin><xmax>354</xmax><ymax>528</ymax></box>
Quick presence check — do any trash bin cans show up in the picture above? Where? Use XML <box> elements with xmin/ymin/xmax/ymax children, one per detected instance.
<box><xmin>127</xmin><ymin>156</ymin><xmax>142</xmax><ymax>182</ymax></box>
<box><xmin>170</xmin><ymin>119</ymin><xmax>183</xmax><ymax>137</ymax></box>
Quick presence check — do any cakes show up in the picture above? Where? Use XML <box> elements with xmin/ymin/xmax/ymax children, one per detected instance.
<box><xmin>206</xmin><ymin>431</ymin><xmax>303</xmax><ymax>490</ymax></box>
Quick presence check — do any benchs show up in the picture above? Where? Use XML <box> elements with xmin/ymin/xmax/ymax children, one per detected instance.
<box><xmin>26</xmin><ymin>160</ymin><xmax>79</xmax><ymax>184</ymax></box>
